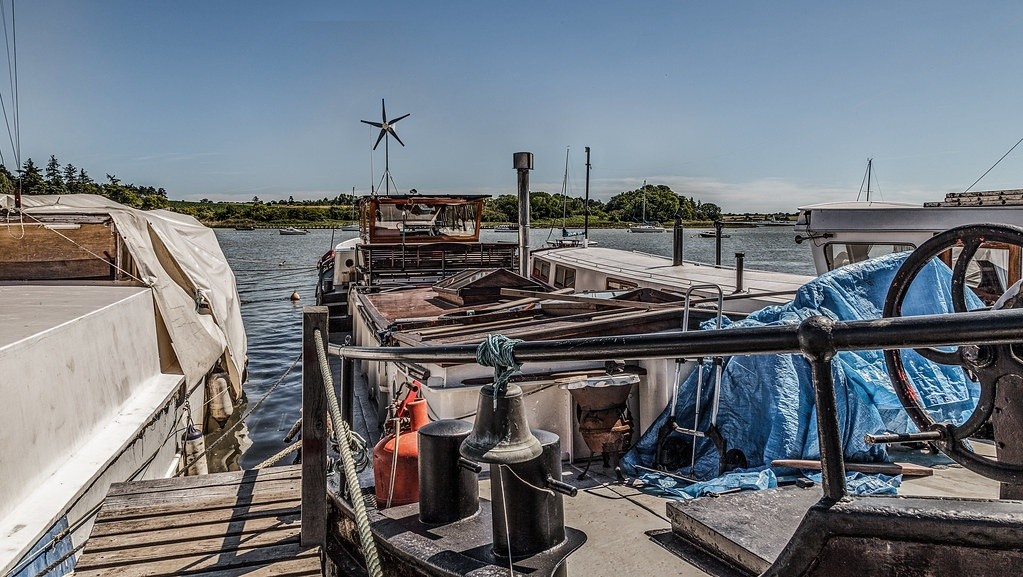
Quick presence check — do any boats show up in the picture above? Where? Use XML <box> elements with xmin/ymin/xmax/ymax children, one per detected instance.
<box><xmin>235</xmin><ymin>225</ymin><xmax>255</xmax><ymax>231</ymax></box>
<box><xmin>513</xmin><ymin>143</ymin><xmax>1023</xmax><ymax>321</ymax></box>
<box><xmin>316</xmin><ymin>189</ymin><xmax>723</xmax><ymax>476</ymax></box>
<box><xmin>0</xmin><ymin>193</ymin><xmax>252</xmax><ymax>577</ymax></box>
<box><xmin>719</xmin><ymin>221</ymin><xmax>759</xmax><ymax>228</ymax></box>
<box><xmin>699</xmin><ymin>230</ymin><xmax>731</xmax><ymax>238</ymax></box>
<box><xmin>768</xmin><ymin>215</ymin><xmax>797</xmax><ymax>226</ymax></box>
<box><xmin>279</xmin><ymin>226</ymin><xmax>307</xmax><ymax>236</ymax></box>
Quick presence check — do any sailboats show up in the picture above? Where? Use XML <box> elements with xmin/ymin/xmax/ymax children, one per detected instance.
<box><xmin>545</xmin><ymin>145</ymin><xmax>599</xmax><ymax>248</ymax></box>
<box><xmin>629</xmin><ymin>179</ymin><xmax>673</xmax><ymax>233</ymax></box>
<box><xmin>342</xmin><ymin>187</ymin><xmax>360</xmax><ymax>231</ymax></box>
